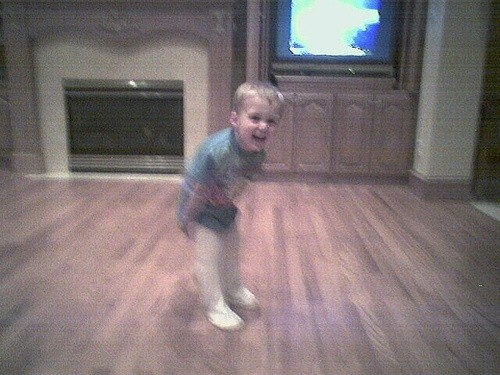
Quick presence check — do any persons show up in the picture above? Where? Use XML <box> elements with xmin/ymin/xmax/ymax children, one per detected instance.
<box><xmin>175</xmin><ymin>79</ymin><xmax>286</xmax><ymax>332</ymax></box>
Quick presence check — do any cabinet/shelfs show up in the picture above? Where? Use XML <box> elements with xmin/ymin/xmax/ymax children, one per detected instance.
<box><xmin>263</xmin><ymin>92</ymin><xmax>332</xmax><ymax>186</ymax></box>
<box><xmin>333</xmin><ymin>90</ymin><xmax>414</xmax><ymax>183</ymax></box>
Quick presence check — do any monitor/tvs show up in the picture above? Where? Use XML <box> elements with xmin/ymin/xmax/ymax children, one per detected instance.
<box><xmin>271</xmin><ymin>0</ymin><xmax>394</xmax><ymax>75</ymax></box>
